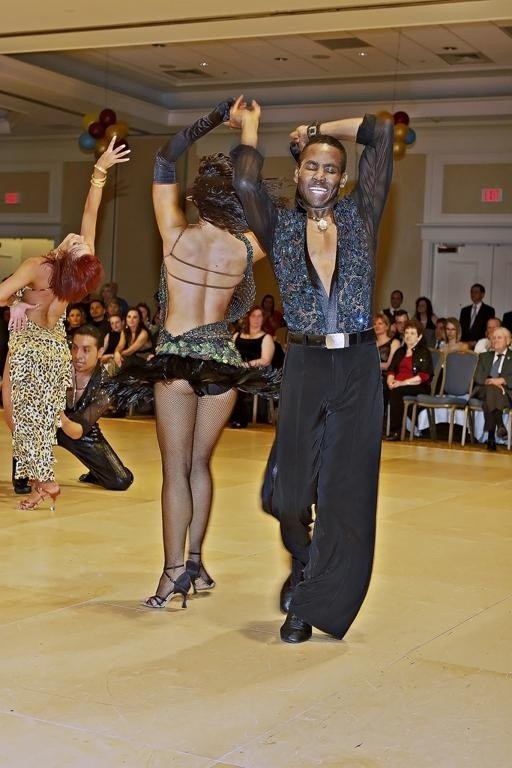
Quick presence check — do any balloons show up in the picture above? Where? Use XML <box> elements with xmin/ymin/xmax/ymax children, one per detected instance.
<box><xmin>78</xmin><ymin>109</ymin><xmax>130</xmax><ymax>161</ymax></box>
<box><xmin>374</xmin><ymin>111</ymin><xmax>416</xmax><ymax>162</ymax></box>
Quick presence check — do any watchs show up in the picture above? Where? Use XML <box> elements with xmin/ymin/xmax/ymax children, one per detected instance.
<box><xmin>306</xmin><ymin>120</ymin><xmax>321</xmax><ymax>142</ymax></box>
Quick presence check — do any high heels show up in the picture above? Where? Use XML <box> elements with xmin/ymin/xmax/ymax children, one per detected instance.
<box><xmin>140</xmin><ymin>564</ymin><xmax>191</xmax><ymax>608</ymax></box>
<box><xmin>185</xmin><ymin>552</ymin><xmax>215</xmax><ymax>594</ymax></box>
<box><xmin>16</xmin><ymin>484</ymin><xmax>60</xmax><ymax>511</ymax></box>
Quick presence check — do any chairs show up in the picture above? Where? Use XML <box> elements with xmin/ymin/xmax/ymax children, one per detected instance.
<box><xmin>409</xmin><ymin>348</ymin><xmax>475</xmax><ymax>445</ymax></box>
<box><xmin>401</xmin><ymin>347</ymin><xmax>445</xmax><ymax>445</ymax></box>
<box><xmin>464</xmin><ymin>391</ymin><xmax>512</xmax><ymax>451</ymax></box>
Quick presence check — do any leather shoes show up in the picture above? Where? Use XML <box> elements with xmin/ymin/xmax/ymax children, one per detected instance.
<box><xmin>15</xmin><ymin>485</ymin><xmax>31</xmax><ymax>494</ymax></box>
<box><xmin>487</xmin><ymin>440</ymin><xmax>496</xmax><ymax>451</ymax></box>
<box><xmin>79</xmin><ymin>472</ymin><xmax>94</xmax><ymax>483</ymax></box>
<box><xmin>497</xmin><ymin>426</ymin><xmax>508</xmax><ymax>440</ymax></box>
<box><xmin>280</xmin><ymin>610</ymin><xmax>312</xmax><ymax>643</ymax></box>
<box><xmin>385</xmin><ymin>432</ymin><xmax>400</xmax><ymax>441</ymax></box>
<box><xmin>280</xmin><ymin>570</ymin><xmax>304</xmax><ymax>613</ymax></box>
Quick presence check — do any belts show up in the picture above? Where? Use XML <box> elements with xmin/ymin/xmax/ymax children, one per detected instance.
<box><xmin>287</xmin><ymin>328</ymin><xmax>377</xmax><ymax>350</ymax></box>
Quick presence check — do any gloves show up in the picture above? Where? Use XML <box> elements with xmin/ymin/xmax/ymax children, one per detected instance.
<box><xmin>152</xmin><ymin>95</ymin><xmax>235</xmax><ymax>183</ymax></box>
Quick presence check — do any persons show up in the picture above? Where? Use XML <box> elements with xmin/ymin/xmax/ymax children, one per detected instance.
<box><xmin>139</xmin><ymin>93</ymin><xmax>308</xmax><ymax>609</ymax></box>
<box><xmin>1</xmin><ymin>134</ymin><xmax>131</xmax><ymax>510</ymax></box>
<box><xmin>224</xmin><ymin>95</ymin><xmax>396</xmax><ymax>645</ymax></box>
<box><xmin>12</xmin><ymin>326</ymin><xmax>133</xmax><ymax>495</ymax></box>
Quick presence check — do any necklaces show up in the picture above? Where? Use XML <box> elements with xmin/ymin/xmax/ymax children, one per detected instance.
<box><xmin>308</xmin><ymin>216</ymin><xmax>330</xmax><ymax>232</ymax></box>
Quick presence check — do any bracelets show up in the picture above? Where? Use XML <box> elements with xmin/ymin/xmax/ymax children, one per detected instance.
<box><xmin>90</xmin><ymin>164</ymin><xmax>108</xmax><ymax>189</ymax></box>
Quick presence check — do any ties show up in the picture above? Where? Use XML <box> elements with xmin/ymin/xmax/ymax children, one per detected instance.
<box><xmin>437</xmin><ymin>340</ymin><xmax>441</xmax><ymax>350</ymax></box>
<box><xmin>469</xmin><ymin>304</ymin><xmax>477</xmax><ymax>329</ymax></box>
<box><xmin>489</xmin><ymin>354</ymin><xmax>502</xmax><ymax>378</ymax></box>
